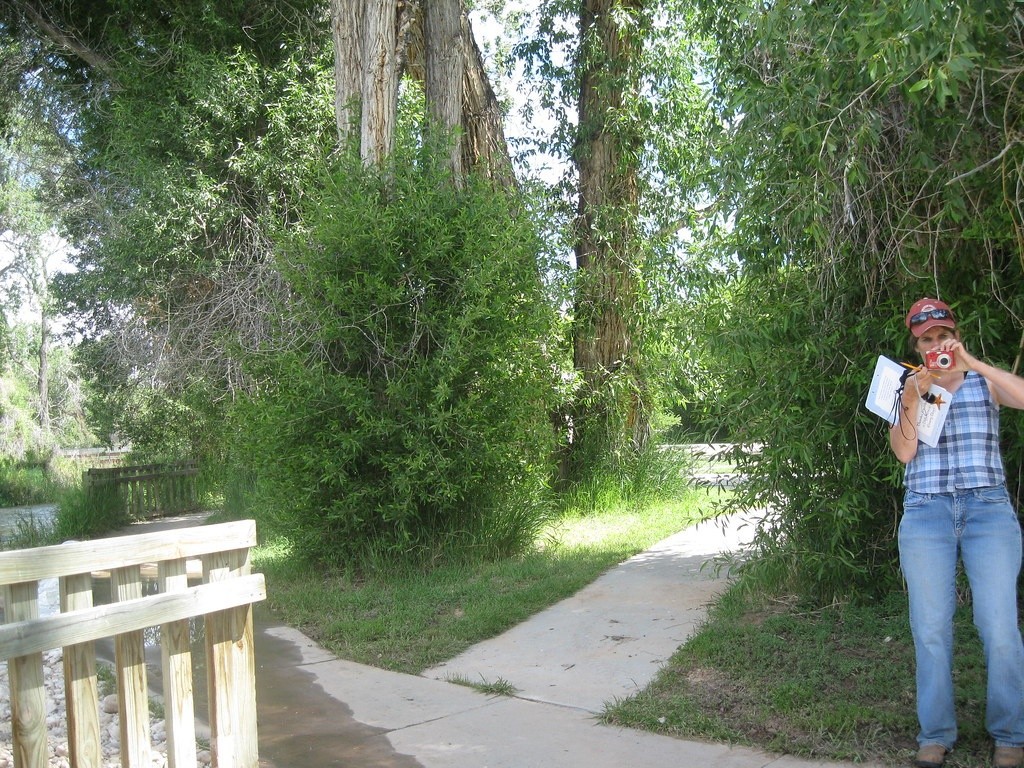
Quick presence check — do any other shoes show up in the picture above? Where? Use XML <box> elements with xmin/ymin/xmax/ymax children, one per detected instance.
<box><xmin>994</xmin><ymin>745</ymin><xmax>1024</xmax><ymax>768</ymax></box>
<box><xmin>916</xmin><ymin>742</ymin><xmax>947</xmax><ymax>768</ymax></box>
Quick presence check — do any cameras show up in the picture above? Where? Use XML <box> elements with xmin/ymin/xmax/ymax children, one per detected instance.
<box><xmin>925</xmin><ymin>350</ymin><xmax>956</xmax><ymax>370</ymax></box>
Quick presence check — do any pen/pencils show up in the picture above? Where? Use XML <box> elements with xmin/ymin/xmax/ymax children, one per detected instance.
<box><xmin>899</xmin><ymin>362</ymin><xmax>942</xmax><ymax>379</ymax></box>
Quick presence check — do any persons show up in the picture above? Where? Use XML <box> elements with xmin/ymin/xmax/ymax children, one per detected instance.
<box><xmin>890</xmin><ymin>297</ymin><xmax>1024</xmax><ymax>768</ymax></box>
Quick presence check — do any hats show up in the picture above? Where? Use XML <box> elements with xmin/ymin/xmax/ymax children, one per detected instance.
<box><xmin>906</xmin><ymin>297</ymin><xmax>957</xmax><ymax>338</ymax></box>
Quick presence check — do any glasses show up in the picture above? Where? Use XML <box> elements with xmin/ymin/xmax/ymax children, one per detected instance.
<box><xmin>908</xmin><ymin>309</ymin><xmax>955</xmax><ymax>334</ymax></box>
